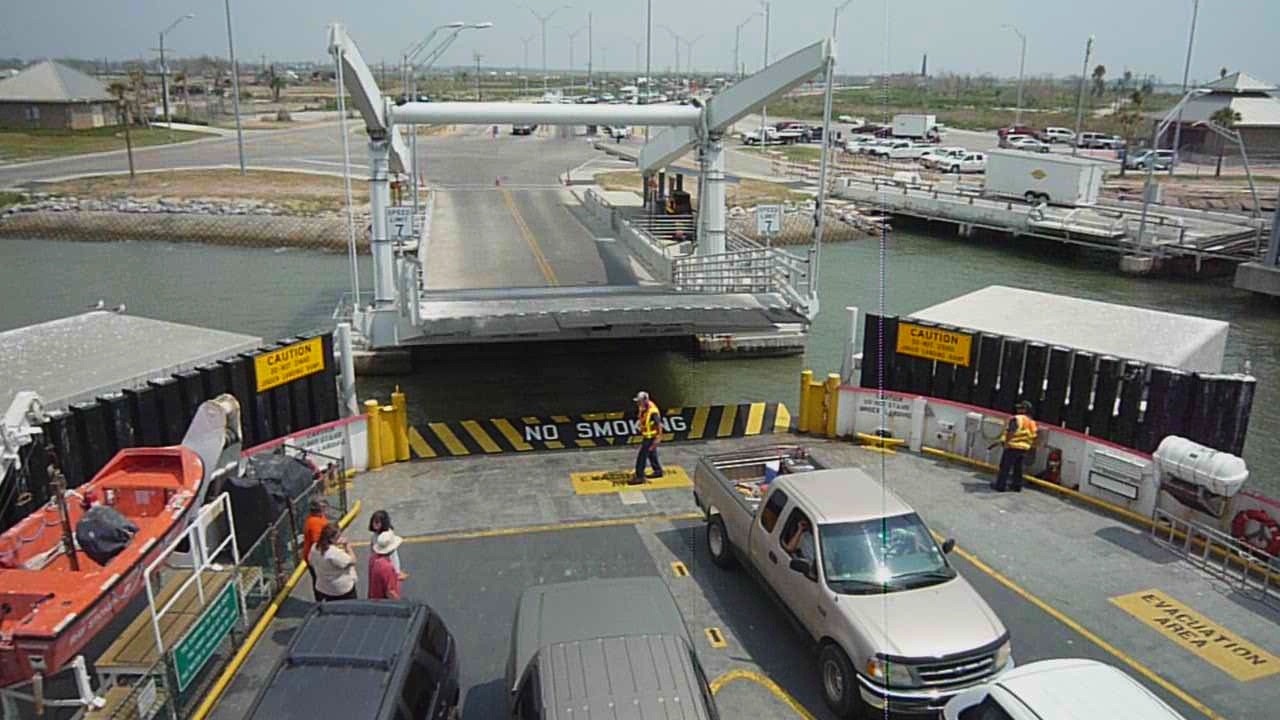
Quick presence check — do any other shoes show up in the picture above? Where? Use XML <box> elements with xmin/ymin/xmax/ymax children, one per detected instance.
<box><xmin>628</xmin><ymin>478</ymin><xmax>643</xmax><ymax>485</ymax></box>
<box><xmin>1008</xmin><ymin>483</ymin><xmax>1021</xmax><ymax>493</ymax></box>
<box><xmin>647</xmin><ymin>472</ymin><xmax>663</xmax><ymax>479</ymax></box>
<box><xmin>989</xmin><ymin>483</ymin><xmax>1005</xmax><ymax>493</ymax></box>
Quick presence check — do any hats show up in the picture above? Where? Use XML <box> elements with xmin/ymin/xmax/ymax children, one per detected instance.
<box><xmin>1016</xmin><ymin>400</ymin><xmax>1032</xmax><ymax>410</ymax></box>
<box><xmin>632</xmin><ymin>391</ymin><xmax>649</xmax><ymax>402</ymax></box>
<box><xmin>372</xmin><ymin>530</ymin><xmax>403</xmax><ymax>554</ymax></box>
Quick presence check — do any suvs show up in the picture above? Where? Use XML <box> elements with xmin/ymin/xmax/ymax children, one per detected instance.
<box><xmin>1126</xmin><ymin>147</ymin><xmax>1179</xmax><ymax>171</ymax></box>
<box><xmin>1070</xmin><ymin>132</ymin><xmax>1127</xmax><ymax>148</ymax></box>
<box><xmin>240</xmin><ymin>597</ymin><xmax>460</xmax><ymax>717</ymax></box>
<box><xmin>503</xmin><ymin>575</ymin><xmax>720</xmax><ymax>720</ymax></box>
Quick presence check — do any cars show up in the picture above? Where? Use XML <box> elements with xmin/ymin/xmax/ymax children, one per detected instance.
<box><xmin>939</xmin><ymin>151</ymin><xmax>988</xmax><ymax>174</ymax></box>
<box><xmin>397</xmin><ymin>93</ymin><xmax>430</xmax><ymax>105</ymax></box>
<box><xmin>513</xmin><ymin>123</ymin><xmax>538</xmax><ymax>135</ymax></box>
<box><xmin>998</xmin><ymin>124</ymin><xmax>1052</xmax><ymax>152</ymax></box>
<box><xmin>940</xmin><ymin>657</ymin><xmax>1188</xmax><ymax>720</ymax></box>
<box><xmin>918</xmin><ymin>145</ymin><xmax>968</xmax><ymax>168</ymax></box>
<box><xmin>741</xmin><ymin>113</ymin><xmax>946</xmax><ymax>158</ymax></box>
<box><xmin>534</xmin><ymin>73</ymin><xmax>728</xmax><ymax>143</ymax></box>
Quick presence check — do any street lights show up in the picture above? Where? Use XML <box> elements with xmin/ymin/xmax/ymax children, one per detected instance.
<box><xmin>530</xmin><ymin>8</ymin><xmax>561</xmax><ymax>93</ymax></box>
<box><xmin>1001</xmin><ymin>23</ymin><xmax>1028</xmax><ymax>125</ymax></box>
<box><xmin>401</xmin><ymin>23</ymin><xmax>464</xmax><ymax>195</ymax></box>
<box><xmin>1129</xmin><ymin>86</ymin><xmax>1217</xmax><ymax>249</ymax></box>
<box><xmin>408</xmin><ymin>22</ymin><xmax>495</xmax><ymax>211</ymax></box>
<box><xmin>158</xmin><ymin>13</ymin><xmax>193</xmax><ymax>124</ymax></box>
<box><xmin>1190</xmin><ymin>120</ymin><xmax>1262</xmax><ymax>218</ymax></box>
<box><xmin>736</xmin><ymin>8</ymin><xmax>768</xmax><ymax>80</ymax></box>
<box><xmin>565</xmin><ymin>5</ymin><xmax>593</xmax><ymax>93</ymax></box>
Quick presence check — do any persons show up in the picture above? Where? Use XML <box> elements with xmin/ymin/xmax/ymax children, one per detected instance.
<box><xmin>368</xmin><ymin>509</ymin><xmax>401</xmax><ymax>572</ymax></box>
<box><xmin>990</xmin><ymin>400</ymin><xmax>1037</xmax><ymax>492</ymax></box>
<box><xmin>367</xmin><ymin>530</ymin><xmax>403</xmax><ymax>600</ymax></box>
<box><xmin>627</xmin><ymin>392</ymin><xmax>663</xmax><ymax>484</ymax></box>
<box><xmin>303</xmin><ymin>495</ymin><xmax>328</xmax><ymax>602</ymax></box>
<box><xmin>785</xmin><ymin>518</ymin><xmax>837</xmax><ymax>576</ymax></box>
<box><xmin>878</xmin><ymin>519</ymin><xmax>920</xmax><ymax>558</ymax></box>
<box><xmin>308</xmin><ymin>523</ymin><xmax>358</xmax><ymax>602</ymax></box>
<box><xmin>491</xmin><ymin>126</ymin><xmax>499</xmax><ymax>143</ymax></box>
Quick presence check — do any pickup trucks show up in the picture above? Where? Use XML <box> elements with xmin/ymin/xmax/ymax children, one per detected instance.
<box><xmin>694</xmin><ymin>444</ymin><xmax>1014</xmax><ymax>718</ymax></box>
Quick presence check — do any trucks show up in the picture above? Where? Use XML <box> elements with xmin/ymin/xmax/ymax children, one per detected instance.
<box><xmin>890</xmin><ymin>113</ymin><xmax>947</xmax><ymax>144</ymax></box>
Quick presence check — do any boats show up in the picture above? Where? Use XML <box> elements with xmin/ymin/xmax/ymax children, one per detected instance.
<box><xmin>0</xmin><ymin>444</ymin><xmax>207</xmax><ymax>689</ymax></box>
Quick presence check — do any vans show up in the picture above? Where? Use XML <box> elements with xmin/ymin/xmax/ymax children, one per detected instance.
<box><xmin>1043</xmin><ymin>127</ymin><xmax>1076</xmax><ymax>143</ymax></box>
